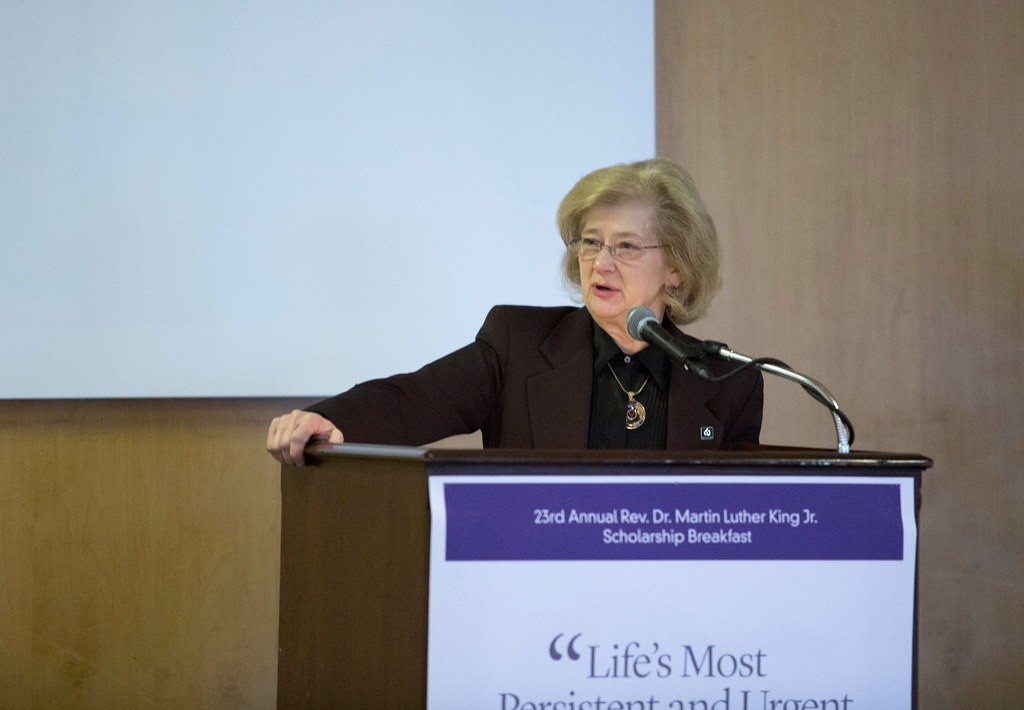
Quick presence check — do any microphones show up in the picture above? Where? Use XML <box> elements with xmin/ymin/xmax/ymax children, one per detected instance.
<box><xmin>624</xmin><ymin>306</ymin><xmax>713</xmax><ymax>382</ymax></box>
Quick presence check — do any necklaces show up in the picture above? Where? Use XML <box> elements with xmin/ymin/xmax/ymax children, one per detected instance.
<box><xmin>596</xmin><ymin>348</ymin><xmax>649</xmax><ymax>431</ymax></box>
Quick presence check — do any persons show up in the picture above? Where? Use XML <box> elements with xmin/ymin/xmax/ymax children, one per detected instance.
<box><xmin>265</xmin><ymin>156</ymin><xmax>766</xmax><ymax>469</ymax></box>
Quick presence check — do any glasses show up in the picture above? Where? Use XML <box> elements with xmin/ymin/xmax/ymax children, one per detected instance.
<box><xmin>569</xmin><ymin>238</ymin><xmax>675</xmax><ymax>262</ymax></box>
